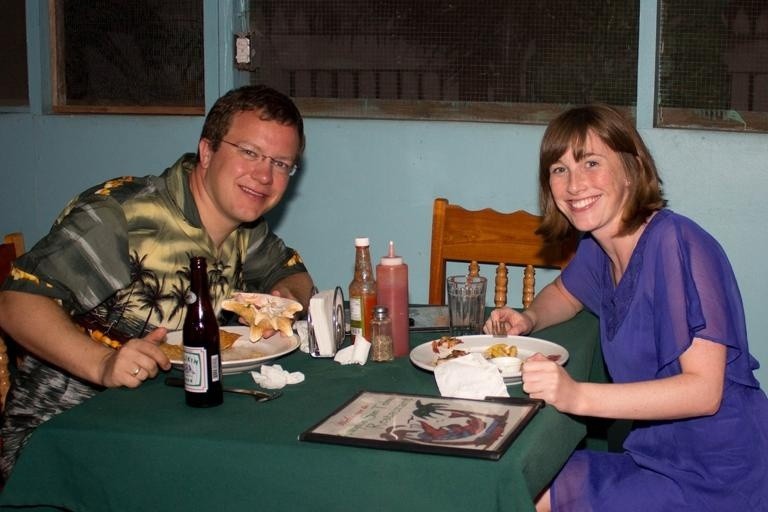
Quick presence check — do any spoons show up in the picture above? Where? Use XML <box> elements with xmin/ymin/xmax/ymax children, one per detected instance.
<box><xmin>164</xmin><ymin>374</ymin><xmax>284</xmax><ymax>404</ymax></box>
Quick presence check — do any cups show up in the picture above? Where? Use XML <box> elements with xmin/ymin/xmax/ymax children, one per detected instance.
<box><xmin>446</xmin><ymin>274</ymin><xmax>487</xmax><ymax>337</ymax></box>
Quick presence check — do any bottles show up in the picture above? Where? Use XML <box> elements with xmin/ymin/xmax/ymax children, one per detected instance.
<box><xmin>350</xmin><ymin>237</ymin><xmax>378</xmax><ymax>345</ymax></box>
<box><xmin>370</xmin><ymin>307</ymin><xmax>394</xmax><ymax>362</ymax></box>
<box><xmin>377</xmin><ymin>241</ymin><xmax>410</xmax><ymax>356</ymax></box>
<box><xmin>183</xmin><ymin>258</ymin><xmax>224</xmax><ymax>408</ymax></box>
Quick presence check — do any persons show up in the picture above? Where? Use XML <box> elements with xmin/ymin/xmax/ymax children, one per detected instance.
<box><xmin>0</xmin><ymin>84</ymin><xmax>317</xmax><ymax>494</ymax></box>
<box><xmin>483</xmin><ymin>105</ymin><xmax>768</xmax><ymax>511</ymax></box>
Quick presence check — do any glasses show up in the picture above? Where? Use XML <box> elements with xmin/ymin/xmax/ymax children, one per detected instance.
<box><xmin>213</xmin><ymin>137</ymin><xmax>298</xmax><ymax>178</ymax></box>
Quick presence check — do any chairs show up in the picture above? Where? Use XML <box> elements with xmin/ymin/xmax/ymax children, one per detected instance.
<box><xmin>0</xmin><ymin>234</ymin><xmax>25</xmax><ymax>413</ymax></box>
<box><xmin>429</xmin><ymin>199</ymin><xmax>586</xmax><ymax>314</ymax></box>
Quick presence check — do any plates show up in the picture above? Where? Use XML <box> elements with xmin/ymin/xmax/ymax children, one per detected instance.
<box><xmin>156</xmin><ymin>325</ymin><xmax>300</xmax><ymax>376</ymax></box>
<box><xmin>409</xmin><ymin>333</ymin><xmax>569</xmax><ymax>387</ymax></box>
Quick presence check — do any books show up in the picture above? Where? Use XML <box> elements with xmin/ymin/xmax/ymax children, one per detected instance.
<box><xmin>298</xmin><ymin>390</ymin><xmax>546</xmax><ymax>461</ymax></box>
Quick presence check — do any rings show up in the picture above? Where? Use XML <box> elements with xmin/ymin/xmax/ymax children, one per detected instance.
<box><xmin>134</xmin><ymin>368</ymin><xmax>143</xmax><ymax>376</ymax></box>
